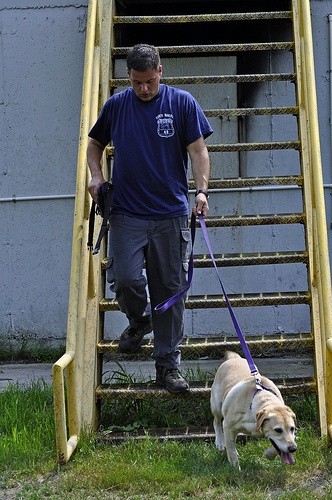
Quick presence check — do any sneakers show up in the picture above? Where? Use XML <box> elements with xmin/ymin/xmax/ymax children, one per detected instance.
<box><xmin>155</xmin><ymin>367</ymin><xmax>190</xmax><ymax>394</ymax></box>
<box><xmin>119</xmin><ymin>315</ymin><xmax>152</xmax><ymax>354</ymax></box>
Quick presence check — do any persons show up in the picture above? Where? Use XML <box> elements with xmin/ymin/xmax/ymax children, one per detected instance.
<box><xmin>87</xmin><ymin>45</ymin><xmax>214</xmax><ymax>395</ymax></box>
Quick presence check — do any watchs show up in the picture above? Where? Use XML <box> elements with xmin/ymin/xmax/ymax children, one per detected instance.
<box><xmin>195</xmin><ymin>189</ymin><xmax>209</xmax><ymax>200</ymax></box>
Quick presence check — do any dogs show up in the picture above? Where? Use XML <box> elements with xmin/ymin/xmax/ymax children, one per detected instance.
<box><xmin>209</xmin><ymin>350</ymin><xmax>298</xmax><ymax>471</ymax></box>
